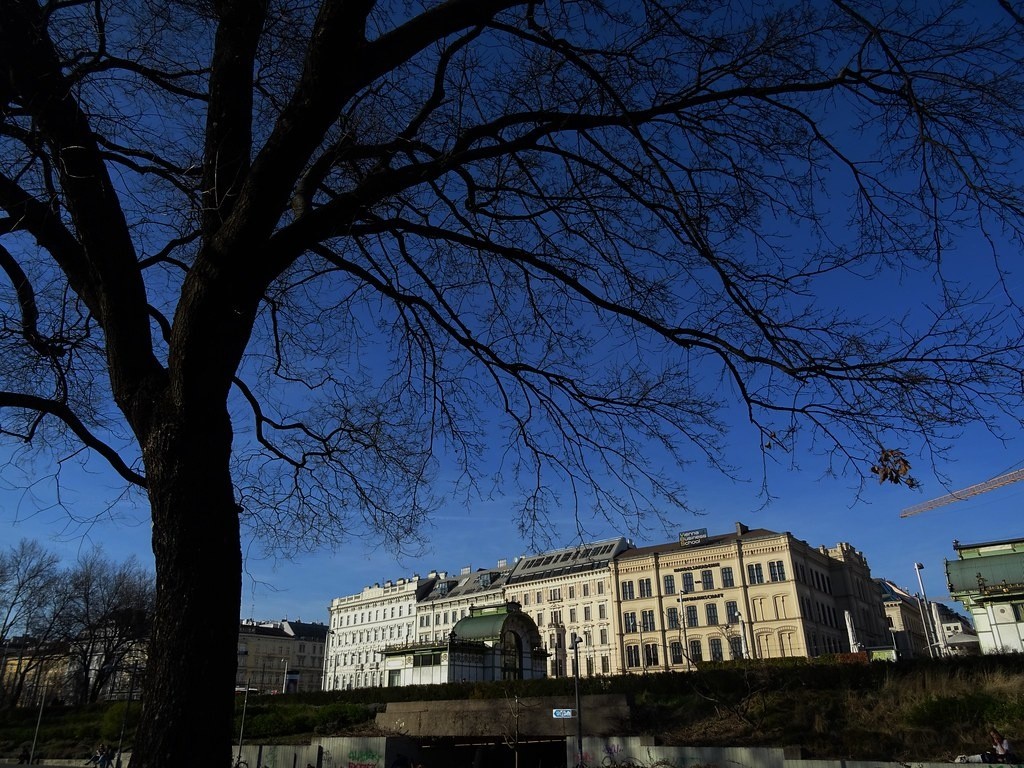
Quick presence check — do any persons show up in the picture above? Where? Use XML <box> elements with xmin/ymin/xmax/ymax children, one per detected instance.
<box><xmin>955</xmin><ymin>729</ymin><xmax>1009</xmax><ymax>763</ymax></box>
<box><xmin>84</xmin><ymin>742</ymin><xmax>120</xmax><ymax>768</ymax></box>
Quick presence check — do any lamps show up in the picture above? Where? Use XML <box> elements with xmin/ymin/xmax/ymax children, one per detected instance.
<box><xmin>1002</xmin><ymin>580</ymin><xmax>1009</xmax><ymax>593</ymax></box>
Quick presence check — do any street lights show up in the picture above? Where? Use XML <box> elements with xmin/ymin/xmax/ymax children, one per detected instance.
<box><xmin>321</xmin><ymin>628</ymin><xmax>335</xmax><ymax>691</ymax></box>
<box><xmin>677</xmin><ymin>589</ymin><xmax>689</xmax><ymax>669</ymax></box>
<box><xmin>281</xmin><ymin>659</ymin><xmax>288</xmax><ymax>694</ymax></box>
<box><xmin>572</xmin><ymin>631</ymin><xmax>583</xmax><ymax>768</ymax></box>
<box><xmin>734</xmin><ymin>611</ymin><xmax>748</xmax><ymax>661</ymax></box>
<box><xmin>914</xmin><ymin>562</ymin><xmax>941</xmax><ymax>658</ymax></box>
